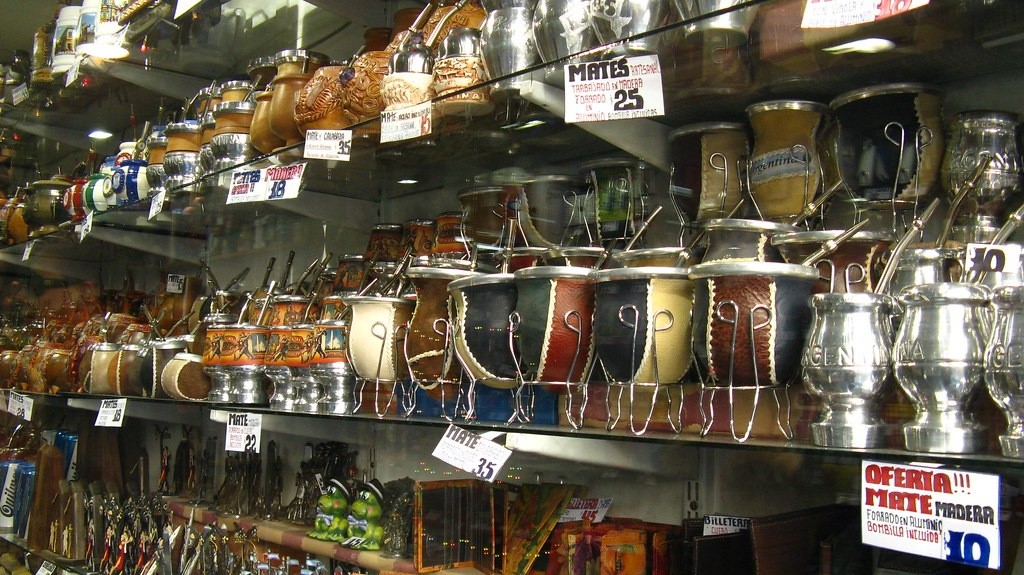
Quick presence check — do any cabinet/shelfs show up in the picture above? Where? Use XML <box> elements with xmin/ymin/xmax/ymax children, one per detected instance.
<box><xmin>0</xmin><ymin>0</ymin><xmax>1024</xmax><ymax>574</ymax></box>
